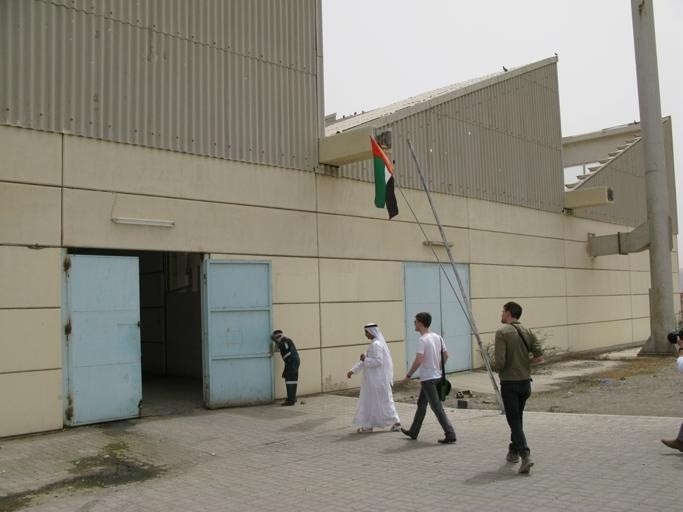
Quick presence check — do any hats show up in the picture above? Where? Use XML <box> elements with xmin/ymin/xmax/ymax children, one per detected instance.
<box><xmin>272</xmin><ymin>330</ymin><xmax>282</xmax><ymax>340</ymax></box>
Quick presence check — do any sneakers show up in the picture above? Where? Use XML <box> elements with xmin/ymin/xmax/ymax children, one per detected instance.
<box><xmin>507</xmin><ymin>452</ymin><xmax>519</xmax><ymax>462</ymax></box>
<box><xmin>520</xmin><ymin>456</ymin><xmax>534</xmax><ymax>472</ymax></box>
<box><xmin>662</xmin><ymin>439</ymin><xmax>683</xmax><ymax>452</ymax></box>
<box><xmin>282</xmin><ymin>398</ymin><xmax>297</xmax><ymax>406</ymax></box>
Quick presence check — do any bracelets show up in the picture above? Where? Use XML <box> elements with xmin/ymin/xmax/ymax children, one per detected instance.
<box><xmin>405</xmin><ymin>374</ymin><xmax>411</xmax><ymax>378</ymax></box>
<box><xmin>678</xmin><ymin>347</ymin><xmax>683</xmax><ymax>352</ymax></box>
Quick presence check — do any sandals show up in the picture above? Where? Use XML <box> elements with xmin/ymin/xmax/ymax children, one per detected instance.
<box><xmin>357</xmin><ymin>427</ymin><xmax>373</xmax><ymax>432</ymax></box>
<box><xmin>391</xmin><ymin>424</ymin><xmax>401</xmax><ymax>431</ymax></box>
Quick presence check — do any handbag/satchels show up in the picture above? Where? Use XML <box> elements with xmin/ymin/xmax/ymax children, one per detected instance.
<box><xmin>438</xmin><ymin>378</ymin><xmax>451</xmax><ymax>400</ymax></box>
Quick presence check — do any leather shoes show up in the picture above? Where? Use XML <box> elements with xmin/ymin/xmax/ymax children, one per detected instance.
<box><xmin>438</xmin><ymin>438</ymin><xmax>456</xmax><ymax>443</ymax></box>
<box><xmin>401</xmin><ymin>428</ymin><xmax>417</xmax><ymax>439</ymax></box>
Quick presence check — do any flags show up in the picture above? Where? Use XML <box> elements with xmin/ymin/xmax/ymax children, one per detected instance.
<box><xmin>370</xmin><ymin>135</ymin><xmax>398</xmax><ymax>219</ymax></box>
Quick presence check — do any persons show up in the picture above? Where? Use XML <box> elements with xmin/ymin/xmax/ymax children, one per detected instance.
<box><xmin>344</xmin><ymin>323</ymin><xmax>400</xmax><ymax>434</ymax></box>
<box><xmin>271</xmin><ymin>329</ymin><xmax>300</xmax><ymax>406</ymax></box>
<box><xmin>489</xmin><ymin>301</ymin><xmax>545</xmax><ymax>473</ymax></box>
<box><xmin>660</xmin><ymin>331</ymin><xmax>683</xmax><ymax>451</ymax></box>
<box><xmin>397</xmin><ymin>312</ymin><xmax>458</xmax><ymax>444</ymax></box>
